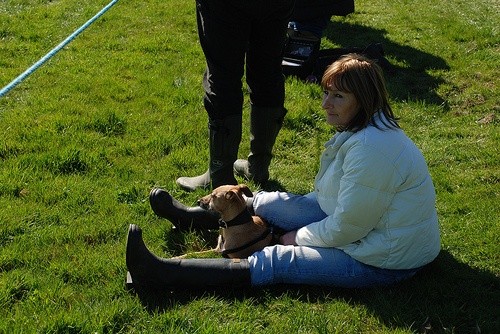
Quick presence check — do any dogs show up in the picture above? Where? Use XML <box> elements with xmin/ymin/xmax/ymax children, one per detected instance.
<box><xmin>168</xmin><ymin>183</ymin><xmax>285</xmax><ymax>259</ymax></box>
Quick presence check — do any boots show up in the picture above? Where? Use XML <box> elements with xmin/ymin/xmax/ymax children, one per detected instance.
<box><xmin>176</xmin><ymin>119</ymin><xmax>240</xmax><ymax>189</ymax></box>
<box><xmin>234</xmin><ymin>122</ymin><xmax>284</xmax><ymax>186</ymax></box>
<box><xmin>126</xmin><ymin>223</ymin><xmax>251</xmax><ymax>317</ymax></box>
<box><xmin>149</xmin><ymin>187</ymin><xmax>262</xmax><ymax>230</ymax></box>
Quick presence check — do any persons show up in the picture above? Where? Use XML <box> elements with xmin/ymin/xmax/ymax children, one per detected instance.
<box><xmin>125</xmin><ymin>53</ymin><xmax>442</xmax><ymax>295</ymax></box>
<box><xmin>175</xmin><ymin>0</ymin><xmax>356</xmax><ymax>191</ymax></box>
